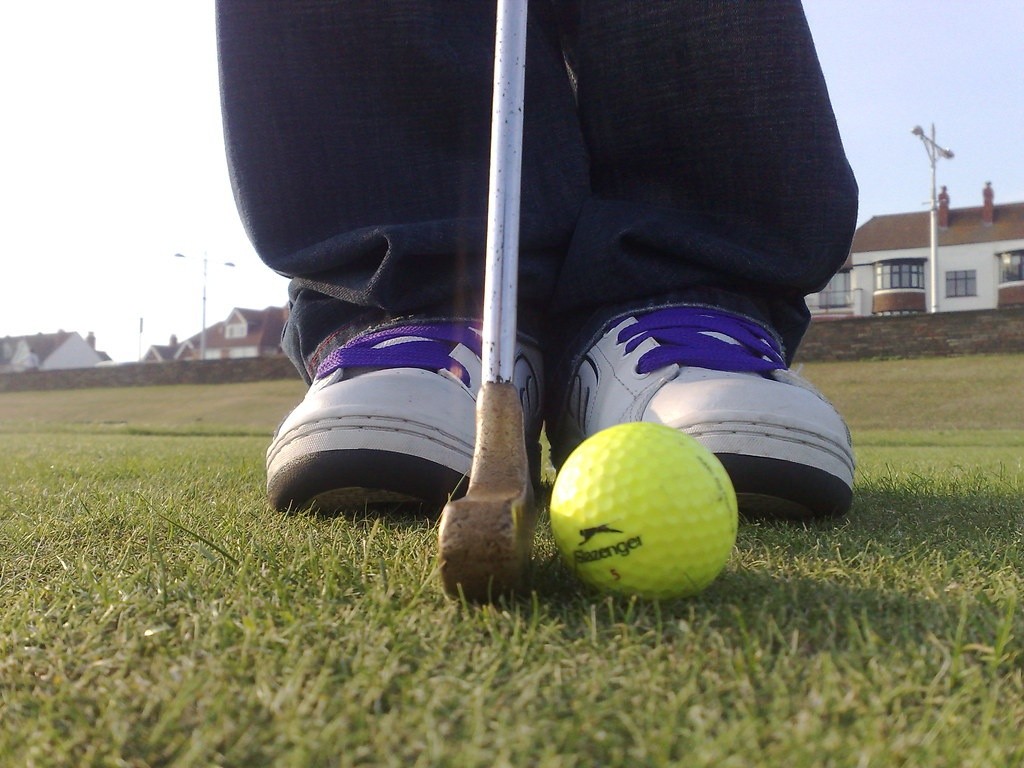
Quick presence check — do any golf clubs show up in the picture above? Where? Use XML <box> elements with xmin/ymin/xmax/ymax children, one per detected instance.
<box><xmin>438</xmin><ymin>0</ymin><xmax>536</xmax><ymax>604</ymax></box>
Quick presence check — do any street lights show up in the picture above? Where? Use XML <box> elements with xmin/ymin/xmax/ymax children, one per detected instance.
<box><xmin>175</xmin><ymin>252</ymin><xmax>236</xmax><ymax>359</ymax></box>
<box><xmin>911</xmin><ymin>122</ymin><xmax>954</xmax><ymax>313</ymax></box>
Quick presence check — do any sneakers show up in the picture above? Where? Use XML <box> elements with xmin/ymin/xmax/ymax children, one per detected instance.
<box><xmin>549</xmin><ymin>305</ymin><xmax>857</xmax><ymax>517</ymax></box>
<box><xmin>266</xmin><ymin>319</ymin><xmax>551</xmax><ymax>511</ymax></box>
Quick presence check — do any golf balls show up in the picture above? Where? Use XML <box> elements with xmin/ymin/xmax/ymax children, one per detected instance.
<box><xmin>549</xmin><ymin>421</ymin><xmax>739</xmax><ymax>604</ymax></box>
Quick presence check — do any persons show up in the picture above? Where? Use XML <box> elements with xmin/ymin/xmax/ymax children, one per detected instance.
<box><xmin>214</xmin><ymin>0</ymin><xmax>860</xmax><ymax>512</ymax></box>
